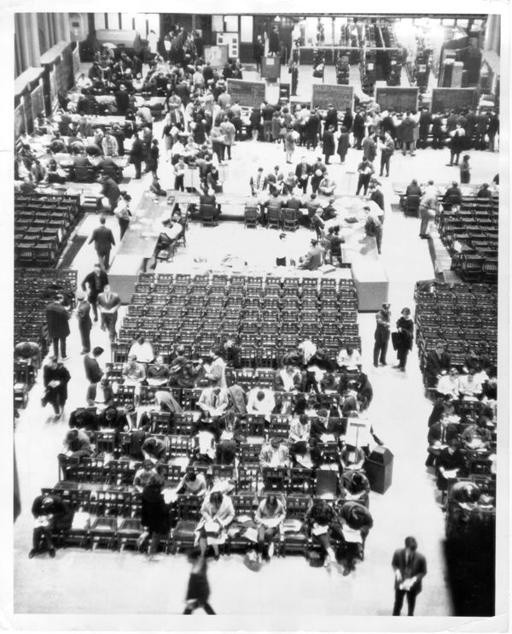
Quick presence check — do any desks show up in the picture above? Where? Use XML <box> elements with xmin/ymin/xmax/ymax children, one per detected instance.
<box><xmin>107</xmin><ymin>189</ymin><xmax>174</xmax><ymax>305</ymax></box>
<box><xmin>175</xmin><ymin>191</ymin><xmax>332</xmax><ymax>210</ymax></box>
<box><xmin>334</xmin><ymin>194</ymin><xmax>389</xmax><ymax>313</ymax></box>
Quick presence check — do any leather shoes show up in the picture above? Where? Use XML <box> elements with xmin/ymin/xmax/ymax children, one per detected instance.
<box><xmin>357</xmin><ymin>551</ymin><xmax>364</xmax><ymax>561</ymax></box>
<box><xmin>343</xmin><ymin>566</ymin><xmax>352</xmax><ymax>576</ymax></box>
<box><xmin>29</xmin><ymin>549</ymin><xmax>36</xmax><ymax>559</ymax></box>
<box><xmin>215</xmin><ymin>555</ymin><xmax>219</xmax><ymax>561</ymax></box>
<box><xmin>48</xmin><ymin>548</ymin><xmax>55</xmax><ymax>558</ymax></box>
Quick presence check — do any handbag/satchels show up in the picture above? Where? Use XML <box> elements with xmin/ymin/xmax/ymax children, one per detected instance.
<box><xmin>40</xmin><ymin>395</ymin><xmax>49</xmax><ymax>408</ymax></box>
<box><xmin>391</xmin><ymin>329</ymin><xmax>402</xmax><ymax>351</ymax></box>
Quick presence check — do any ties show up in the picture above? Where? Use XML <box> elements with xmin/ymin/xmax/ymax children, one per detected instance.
<box><xmin>442</xmin><ymin>425</ymin><xmax>446</xmax><ymax>444</ymax></box>
<box><xmin>406</xmin><ymin>554</ymin><xmax>413</xmax><ymax>569</ymax></box>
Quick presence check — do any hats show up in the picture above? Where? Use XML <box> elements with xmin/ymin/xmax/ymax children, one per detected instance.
<box><xmin>42</xmin><ymin>496</ymin><xmax>54</xmax><ymax>508</ymax></box>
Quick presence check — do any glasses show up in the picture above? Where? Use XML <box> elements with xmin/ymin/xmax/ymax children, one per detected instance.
<box><xmin>318</xmin><ymin>416</ymin><xmax>325</xmax><ymax>421</ymax></box>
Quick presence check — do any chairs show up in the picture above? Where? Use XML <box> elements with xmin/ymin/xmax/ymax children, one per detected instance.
<box><xmin>267</xmin><ymin>206</ymin><xmax>281</xmax><ymax>229</ymax></box>
<box><xmin>176</xmin><ymin>221</ymin><xmax>187</xmax><ymax>248</ymax></box>
<box><xmin>245</xmin><ymin>207</ymin><xmax>258</xmax><ymax>229</ymax></box>
<box><xmin>284</xmin><ymin>207</ymin><xmax>298</xmax><ymax>230</ymax></box>
<box><xmin>113</xmin><ymin>272</ymin><xmax>367</xmax><ymax>363</ymax></box>
<box><xmin>200</xmin><ymin>204</ymin><xmax>218</xmax><ymax>227</ymax></box>
<box><xmin>415</xmin><ymin>282</ymin><xmax>498</xmax><ymax>554</ymax></box>
<box><xmin>14</xmin><ymin>60</ymin><xmax>167</xmax><ymax>193</ymax></box>
<box><xmin>15</xmin><ymin>192</ymin><xmax>84</xmax><ymax>266</ymax></box>
<box><xmin>435</xmin><ymin>195</ymin><xmax>498</xmax><ymax>282</ymax></box>
<box><xmin>14</xmin><ymin>266</ymin><xmax>77</xmax><ymax>421</ymax></box>
<box><xmin>41</xmin><ymin>362</ymin><xmax>370</xmax><ymax>561</ymax></box>
<box><xmin>155</xmin><ymin>239</ymin><xmax>175</xmax><ymax>263</ymax></box>
